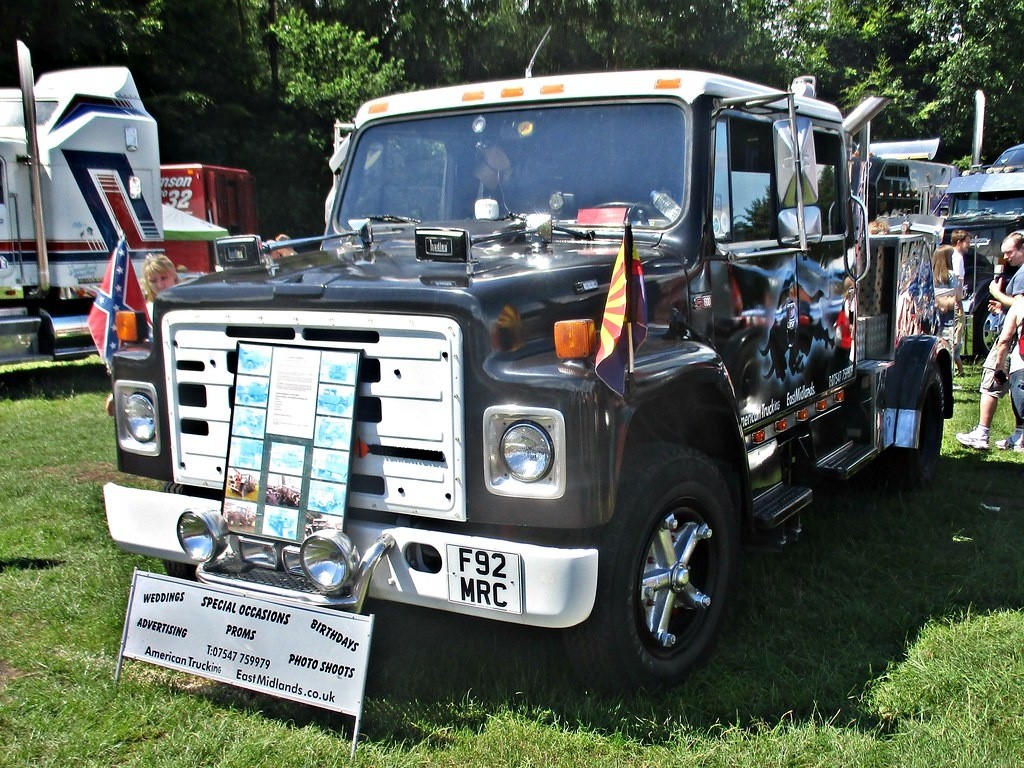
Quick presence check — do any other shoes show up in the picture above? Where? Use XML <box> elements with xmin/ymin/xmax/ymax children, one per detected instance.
<box><xmin>957</xmin><ymin>372</ymin><xmax>966</xmax><ymax>378</ymax></box>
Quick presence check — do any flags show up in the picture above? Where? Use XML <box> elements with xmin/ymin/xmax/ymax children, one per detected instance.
<box><xmin>594</xmin><ymin>232</ymin><xmax>649</xmax><ymax>401</ymax></box>
<box><xmin>86</xmin><ymin>232</ymin><xmax>156</xmax><ymax>378</ymax></box>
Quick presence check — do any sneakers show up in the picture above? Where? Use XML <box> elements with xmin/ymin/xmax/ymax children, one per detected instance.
<box><xmin>956</xmin><ymin>427</ymin><xmax>989</xmax><ymax>451</ymax></box>
<box><xmin>995</xmin><ymin>435</ymin><xmax>1023</xmax><ymax>452</ymax></box>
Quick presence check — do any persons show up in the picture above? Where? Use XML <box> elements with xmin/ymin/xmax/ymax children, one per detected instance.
<box><xmin>106</xmin><ymin>251</ymin><xmax>180</xmax><ymax>418</ymax></box>
<box><xmin>867</xmin><ymin>219</ymin><xmax>891</xmax><ymax>236</ymax></box>
<box><xmin>267</xmin><ymin>233</ymin><xmax>300</xmax><ymax>263</ymax></box>
<box><xmin>932</xmin><ymin>226</ymin><xmax>1024</xmax><ymax>453</ymax></box>
<box><xmin>830</xmin><ymin>275</ymin><xmax>857</xmax><ymax>372</ymax></box>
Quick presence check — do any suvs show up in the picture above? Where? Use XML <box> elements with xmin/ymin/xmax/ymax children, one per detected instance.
<box><xmin>102</xmin><ymin>69</ymin><xmax>957</xmax><ymax>695</ymax></box>
<box><xmin>867</xmin><ymin>143</ymin><xmax>1024</xmax><ymax>360</ymax></box>
